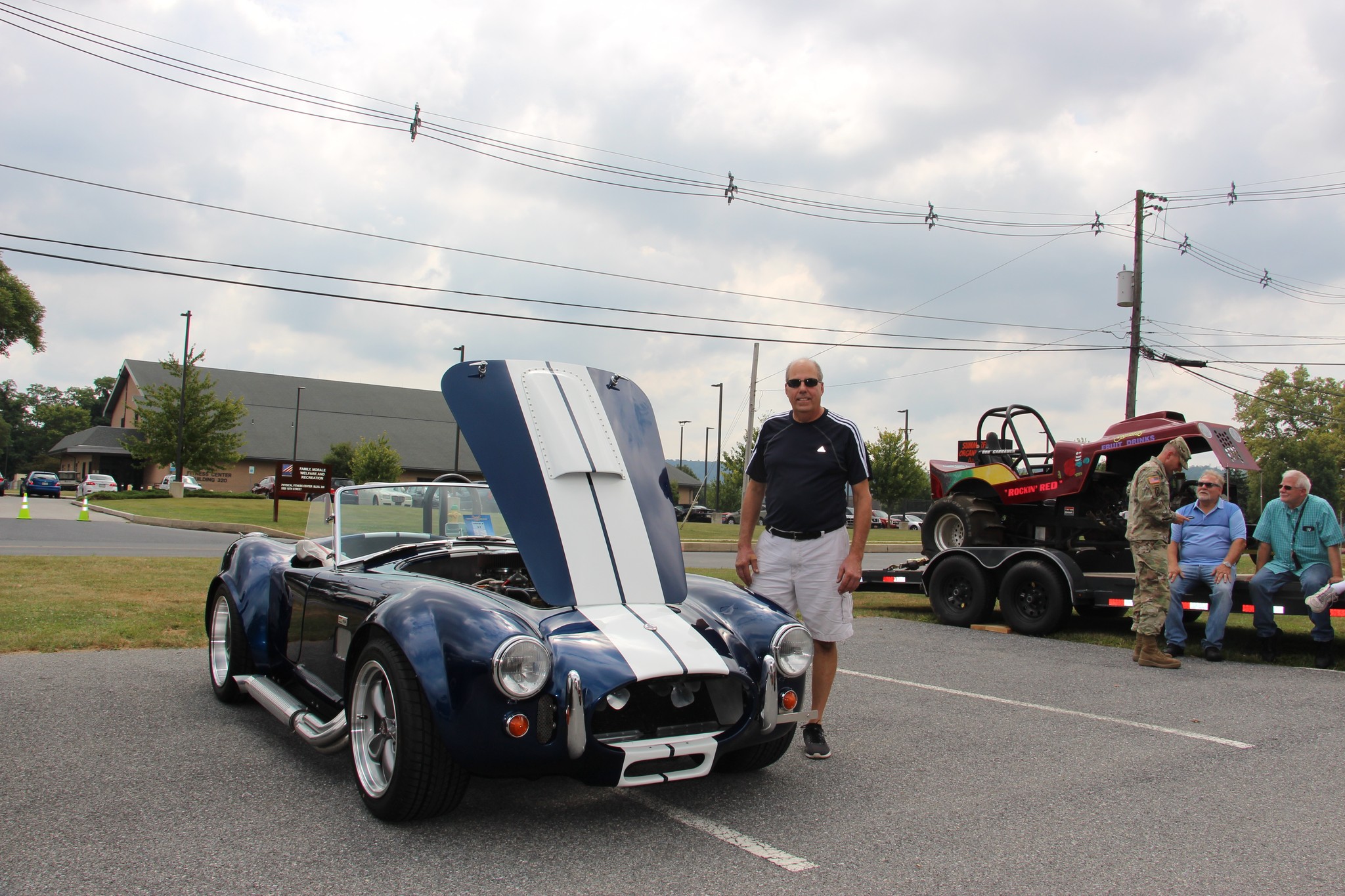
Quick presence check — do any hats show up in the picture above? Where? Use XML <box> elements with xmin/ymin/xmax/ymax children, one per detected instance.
<box><xmin>1170</xmin><ymin>436</ymin><xmax>1191</xmax><ymax>470</ymax></box>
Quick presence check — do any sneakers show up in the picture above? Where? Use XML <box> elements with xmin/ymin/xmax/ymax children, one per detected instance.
<box><xmin>1305</xmin><ymin>582</ymin><xmax>1339</xmax><ymax>613</ymax></box>
<box><xmin>800</xmin><ymin>720</ymin><xmax>831</xmax><ymax>759</ymax></box>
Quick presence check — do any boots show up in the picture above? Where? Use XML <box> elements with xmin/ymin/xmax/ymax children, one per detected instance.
<box><xmin>1132</xmin><ymin>632</ymin><xmax>1181</xmax><ymax>667</ymax></box>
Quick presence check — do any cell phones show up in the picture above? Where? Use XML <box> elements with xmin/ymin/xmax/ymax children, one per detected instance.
<box><xmin>1185</xmin><ymin>516</ymin><xmax>1194</xmax><ymax>519</ymax></box>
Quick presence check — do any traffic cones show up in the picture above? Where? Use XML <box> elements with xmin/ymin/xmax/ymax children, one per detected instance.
<box><xmin>77</xmin><ymin>496</ymin><xmax>92</xmax><ymax>522</ymax></box>
<box><xmin>15</xmin><ymin>493</ymin><xmax>32</xmax><ymax>520</ymax></box>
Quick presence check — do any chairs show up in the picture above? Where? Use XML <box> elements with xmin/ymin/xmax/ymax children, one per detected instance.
<box><xmin>980</xmin><ymin>432</ymin><xmax>1020</xmax><ymax>476</ymax></box>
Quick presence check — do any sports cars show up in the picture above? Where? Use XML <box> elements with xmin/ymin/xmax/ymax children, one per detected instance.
<box><xmin>205</xmin><ymin>357</ymin><xmax>818</xmax><ymax>828</ymax></box>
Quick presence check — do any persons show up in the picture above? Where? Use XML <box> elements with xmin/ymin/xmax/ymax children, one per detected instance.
<box><xmin>1125</xmin><ymin>435</ymin><xmax>1191</xmax><ymax>669</ymax></box>
<box><xmin>1305</xmin><ymin>580</ymin><xmax>1345</xmax><ymax>613</ymax></box>
<box><xmin>1162</xmin><ymin>469</ymin><xmax>1246</xmax><ymax>663</ymax></box>
<box><xmin>1249</xmin><ymin>470</ymin><xmax>1345</xmax><ymax>669</ymax></box>
<box><xmin>736</xmin><ymin>358</ymin><xmax>872</xmax><ymax>758</ymax></box>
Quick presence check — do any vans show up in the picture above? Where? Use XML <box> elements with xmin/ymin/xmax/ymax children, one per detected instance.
<box><xmin>57</xmin><ymin>471</ymin><xmax>81</xmax><ymax>491</ymax></box>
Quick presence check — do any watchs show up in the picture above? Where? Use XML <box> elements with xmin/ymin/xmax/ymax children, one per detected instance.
<box><xmin>1221</xmin><ymin>561</ymin><xmax>1232</xmax><ymax>569</ymax></box>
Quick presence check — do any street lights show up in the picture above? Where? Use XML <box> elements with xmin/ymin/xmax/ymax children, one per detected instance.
<box><xmin>293</xmin><ymin>386</ymin><xmax>306</xmax><ymax>462</ymax></box>
<box><xmin>677</xmin><ymin>421</ymin><xmax>692</xmax><ymax>471</ymax></box>
<box><xmin>710</xmin><ymin>383</ymin><xmax>723</xmax><ymax>513</ymax></box>
<box><xmin>704</xmin><ymin>427</ymin><xmax>715</xmax><ymax>507</ymax></box>
<box><xmin>453</xmin><ymin>345</ymin><xmax>465</xmax><ymax>474</ymax></box>
<box><xmin>897</xmin><ymin>409</ymin><xmax>909</xmax><ymax>522</ymax></box>
<box><xmin>175</xmin><ymin>310</ymin><xmax>193</xmax><ymax>482</ymax></box>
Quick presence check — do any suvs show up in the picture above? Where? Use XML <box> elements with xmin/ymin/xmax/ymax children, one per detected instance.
<box><xmin>356</xmin><ymin>481</ymin><xmax>502</xmax><ymax>514</ymax></box>
<box><xmin>845</xmin><ymin>507</ymin><xmax>882</xmax><ymax>529</ymax></box>
<box><xmin>159</xmin><ymin>474</ymin><xmax>202</xmax><ymax>492</ymax></box>
<box><xmin>872</xmin><ymin>510</ymin><xmax>901</xmax><ymax>529</ymax></box>
<box><xmin>306</xmin><ymin>477</ymin><xmax>359</xmax><ymax>505</ymax></box>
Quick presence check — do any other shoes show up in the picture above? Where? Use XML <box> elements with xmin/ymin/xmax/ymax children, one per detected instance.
<box><xmin>1314</xmin><ymin>642</ymin><xmax>1332</xmax><ymax>668</ymax></box>
<box><xmin>1262</xmin><ymin>628</ymin><xmax>1283</xmax><ymax>662</ymax></box>
<box><xmin>1161</xmin><ymin>643</ymin><xmax>1185</xmax><ymax>657</ymax></box>
<box><xmin>1205</xmin><ymin>647</ymin><xmax>1221</xmax><ymax>661</ymax></box>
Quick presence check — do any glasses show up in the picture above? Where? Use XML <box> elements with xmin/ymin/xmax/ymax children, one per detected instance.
<box><xmin>786</xmin><ymin>378</ymin><xmax>823</xmax><ymax>388</ymax></box>
<box><xmin>1277</xmin><ymin>483</ymin><xmax>1299</xmax><ymax>490</ymax></box>
<box><xmin>1195</xmin><ymin>481</ymin><xmax>1220</xmax><ymax>489</ymax></box>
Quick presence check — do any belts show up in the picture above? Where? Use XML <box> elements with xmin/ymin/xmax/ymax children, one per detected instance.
<box><xmin>762</xmin><ymin>525</ymin><xmax>838</xmax><ymax>541</ymax></box>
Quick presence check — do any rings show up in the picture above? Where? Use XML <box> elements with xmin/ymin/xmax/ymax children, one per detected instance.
<box><xmin>1224</xmin><ymin>574</ymin><xmax>1227</xmax><ymax>576</ymax></box>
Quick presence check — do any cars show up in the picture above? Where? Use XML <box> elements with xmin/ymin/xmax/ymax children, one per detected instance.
<box><xmin>76</xmin><ymin>474</ymin><xmax>117</xmax><ymax>499</ymax></box>
<box><xmin>20</xmin><ymin>471</ymin><xmax>61</xmax><ymax>499</ymax></box>
<box><xmin>725</xmin><ymin>504</ymin><xmax>767</xmax><ymax>526</ymax></box>
<box><xmin>0</xmin><ymin>472</ymin><xmax>9</xmax><ymax>497</ymax></box>
<box><xmin>721</xmin><ymin>512</ymin><xmax>730</xmax><ymax>524</ymax></box>
<box><xmin>250</xmin><ymin>476</ymin><xmax>306</xmax><ymax>501</ymax></box>
<box><xmin>890</xmin><ymin>511</ymin><xmax>927</xmax><ymax>531</ymax></box>
<box><xmin>673</xmin><ymin>505</ymin><xmax>716</xmax><ymax>523</ymax></box>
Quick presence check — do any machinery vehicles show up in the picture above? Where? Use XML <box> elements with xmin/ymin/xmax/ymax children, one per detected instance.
<box><xmin>919</xmin><ymin>404</ymin><xmax>1263</xmax><ymax>639</ymax></box>
